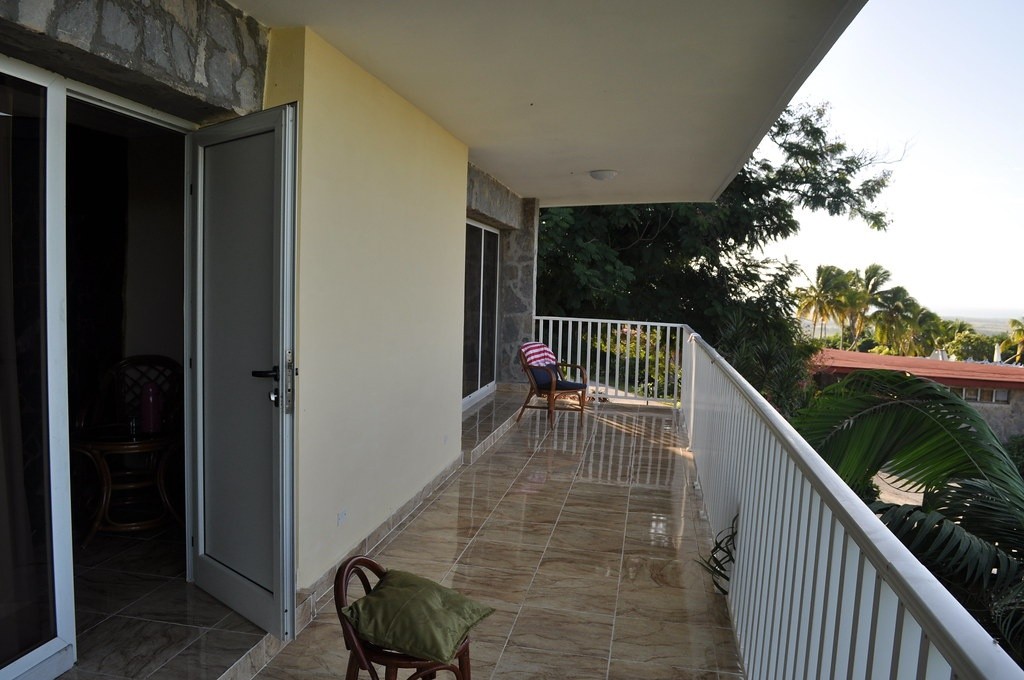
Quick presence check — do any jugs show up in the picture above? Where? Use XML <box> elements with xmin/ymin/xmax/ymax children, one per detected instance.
<box><xmin>140</xmin><ymin>384</ymin><xmax>161</xmax><ymax>432</ymax></box>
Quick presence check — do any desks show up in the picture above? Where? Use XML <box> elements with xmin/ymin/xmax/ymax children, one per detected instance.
<box><xmin>70</xmin><ymin>429</ymin><xmax>186</xmax><ymax>550</ymax></box>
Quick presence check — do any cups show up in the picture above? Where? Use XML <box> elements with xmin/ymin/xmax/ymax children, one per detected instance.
<box><xmin>128</xmin><ymin>416</ymin><xmax>139</xmax><ymax>438</ymax></box>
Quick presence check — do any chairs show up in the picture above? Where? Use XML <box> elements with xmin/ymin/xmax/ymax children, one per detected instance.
<box><xmin>516</xmin><ymin>339</ymin><xmax>588</xmax><ymax>427</ymax></box>
<box><xmin>83</xmin><ymin>352</ymin><xmax>183</xmax><ymax>506</ymax></box>
<box><xmin>335</xmin><ymin>555</ymin><xmax>470</xmax><ymax>680</ymax></box>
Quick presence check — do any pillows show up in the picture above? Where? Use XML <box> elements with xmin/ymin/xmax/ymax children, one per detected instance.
<box><xmin>341</xmin><ymin>569</ymin><xmax>496</xmax><ymax>661</ymax></box>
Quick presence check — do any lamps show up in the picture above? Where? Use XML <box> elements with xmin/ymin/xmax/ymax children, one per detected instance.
<box><xmin>590</xmin><ymin>171</ymin><xmax>616</xmax><ymax>181</ymax></box>
